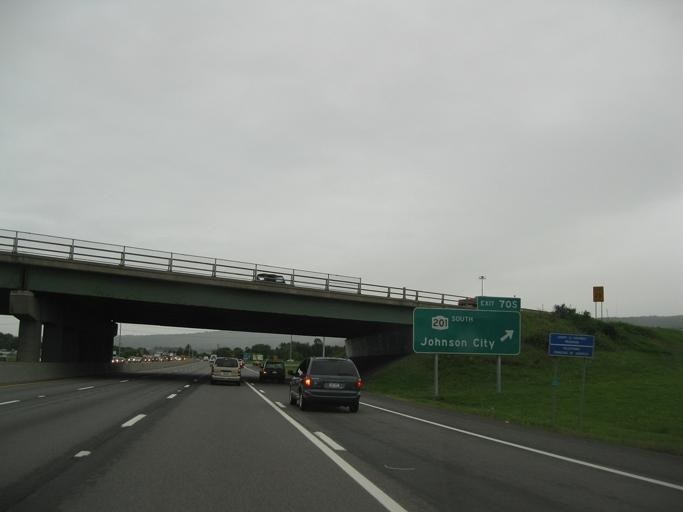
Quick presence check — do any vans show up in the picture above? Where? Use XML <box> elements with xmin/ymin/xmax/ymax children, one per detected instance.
<box><xmin>204</xmin><ymin>355</ymin><xmax>245</xmax><ymax>385</ymax></box>
<box><xmin>259</xmin><ymin>360</ymin><xmax>285</xmax><ymax>382</ymax></box>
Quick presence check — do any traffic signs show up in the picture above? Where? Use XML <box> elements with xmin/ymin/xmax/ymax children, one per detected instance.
<box><xmin>413</xmin><ymin>296</ymin><xmax>521</xmax><ymax>356</ymax></box>
<box><xmin>549</xmin><ymin>333</ymin><xmax>593</xmax><ymax>358</ymax></box>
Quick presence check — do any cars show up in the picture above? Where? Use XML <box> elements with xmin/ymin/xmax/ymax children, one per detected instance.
<box><xmin>289</xmin><ymin>357</ymin><xmax>363</xmax><ymax>412</ymax></box>
<box><xmin>257</xmin><ymin>274</ymin><xmax>285</xmax><ymax>283</ymax></box>
<box><xmin>112</xmin><ymin>356</ymin><xmax>184</xmax><ymax>365</ymax></box>
